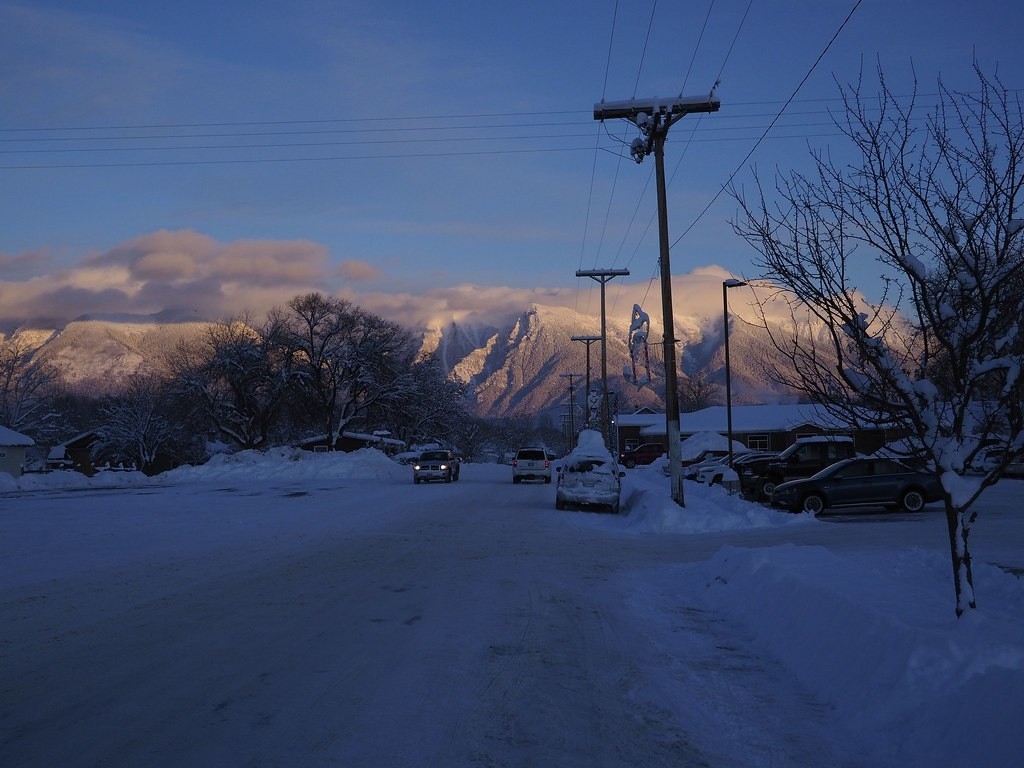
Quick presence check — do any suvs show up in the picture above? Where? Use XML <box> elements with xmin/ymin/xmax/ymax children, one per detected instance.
<box><xmin>617</xmin><ymin>443</ymin><xmax>668</xmax><ymax>468</ymax></box>
<box><xmin>554</xmin><ymin>447</ymin><xmax>626</xmax><ymax>514</ymax></box>
<box><xmin>512</xmin><ymin>447</ymin><xmax>552</xmax><ymax>485</ymax></box>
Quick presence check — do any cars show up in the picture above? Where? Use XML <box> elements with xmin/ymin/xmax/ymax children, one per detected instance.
<box><xmin>770</xmin><ymin>458</ymin><xmax>943</xmax><ymax>517</ymax></box>
<box><xmin>547</xmin><ymin>449</ymin><xmax>561</xmax><ymax>461</ymax></box>
<box><xmin>970</xmin><ymin>445</ymin><xmax>1005</xmax><ymax>474</ymax></box>
<box><xmin>662</xmin><ymin>450</ymin><xmax>780</xmax><ymax>487</ymax></box>
<box><xmin>983</xmin><ymin>445</ymin><xmax>1024</xmax><ymax>478</ymax></box>
<box><xmin>497</xmin><ymin>453</ymin><xmax>515</xmax><ymax>465</ymax></box>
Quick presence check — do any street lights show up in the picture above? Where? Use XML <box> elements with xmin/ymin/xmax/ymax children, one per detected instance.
<box><xmin>606</xmin><ymin>391</ymin><xmax>615</xmax><ymax>458</ymax></box>
<box><xmin>722</xmin><ymin>278</ymin><xmax>748</xmax><ymax>469</ymax></box>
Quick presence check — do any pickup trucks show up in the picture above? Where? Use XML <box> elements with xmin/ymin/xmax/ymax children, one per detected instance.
<box><xmin>413</xmin><ymin>449</ymin><xmax>460</xmax><ymax>484</ymax></box>
<box><xmin>738</xmin><ymin>436</ymin><xmax>929</xmax><ymax>504</ymax></box>
